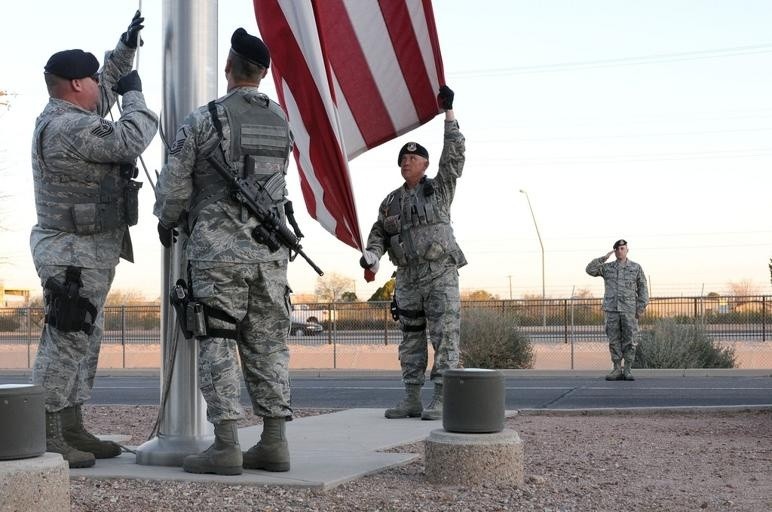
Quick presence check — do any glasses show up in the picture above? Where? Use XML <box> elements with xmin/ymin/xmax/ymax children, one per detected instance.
<box><xmin>91</xmin><ymin>72</ymin><xmax>100</xmax><ymax>79</ymax></box>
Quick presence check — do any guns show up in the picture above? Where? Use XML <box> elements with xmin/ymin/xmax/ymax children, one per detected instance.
<box><xmin>170</xmin><ymin>285</ymin><xmax>191</xmax><ymax>341</ymax></box>
<box><xmin>204</xmin><ymin>143</ymin><xmax>324</xmax><ymax>278</ymax></box>
<box><xmin>44</xmin><ymin>277</ymin><xmax>81</xmax><ymax>332</ymax></box>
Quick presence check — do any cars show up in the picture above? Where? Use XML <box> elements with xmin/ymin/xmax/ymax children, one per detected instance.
<box><xmin>290</xmin><ymin>321</ymin><xmax>323</xmax><ymax>336</ymax></box>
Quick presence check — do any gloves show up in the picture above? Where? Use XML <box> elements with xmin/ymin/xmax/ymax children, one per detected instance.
<box><xmin>111</xmin><ymin>70</ymin><xmax>142</xmax><ymax>95</ymax></box>
<box><xmin>360</xmin><ymin>255</ymin><xmax>375</xmax><ymax>269</ymax></box>
<box><xmin>126</xmin><ymin>10</ymin><xmax>144</xmax><ymax>47</ymax></box>
<box><xmin>440</xmin><ymin>85</ymin><xmax>454</xmax><ymax>110</ymax></box>
<box><xmin>158</xmin><ymin>221</ymin><xmax>179</xmax><ymax>247</ymax></box>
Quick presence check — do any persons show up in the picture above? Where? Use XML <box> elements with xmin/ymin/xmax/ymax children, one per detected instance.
<box><xmin>151</xmin><ymin>26</ymin><xmax>294</xmax><ymax>475</ymax></box>
<box><xmin>363</xmin><ymin>85</ymin><xmax>469</xmax><ymax>420</ymax></box>
<box><xmin>29</xmin><ymin>10</ymin><xmax>160</xmax><ymax>468</ymax></box>
<box><xmin>586</xmin><ymin>240</ymin><xmax>649</xmax><ymax>380</ymax></box>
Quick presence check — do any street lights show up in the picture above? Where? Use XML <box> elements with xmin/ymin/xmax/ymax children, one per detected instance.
<box><xmin>517</xmin><ymin>188</ymin><xmax>549</xmax><ymax>328</ymax></box>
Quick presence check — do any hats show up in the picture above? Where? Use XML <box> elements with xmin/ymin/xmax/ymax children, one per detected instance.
<box><xmin>44</xmin><ymin>49</ymin><xmax>99</xmax><ymax>79</ymax></box>
<box><xmin>398</xmin><ymin>142</ymin><xmax>428</xmax><ymax>167</ymax></box>
<box><xmin>230</xmin><ymin>28</ymin><xmax>270</xmax><ymax>70</ymax></box>
<box><xmin>613</xmin><ymin>240</ymin><xmax>627</xmax><ymax>249</ymax></box>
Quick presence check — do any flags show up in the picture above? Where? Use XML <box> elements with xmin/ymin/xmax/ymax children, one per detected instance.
<box><xmin>253</xmin><ymin>1</ymin><xmax>449</xmax><ymax>255</ymax></box>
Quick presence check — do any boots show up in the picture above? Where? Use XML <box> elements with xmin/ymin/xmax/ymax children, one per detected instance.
<box><xmin>623</xmin><ymin>361</ymin><xmax>635</xmax><ymax>379</ymax></box>
<box><xmin>46</xmin><ymin>404</ymin><xmax>121</xmax><ymax>468</ymax></box>
<box><xmin>242</xmin><ymin>416</ymin><xmax>290</xmax><ymax>472</ymax></box>
<box><xmin>385</xmin><ymin>384</ymin><xmax>424</xmax><ymax>418</ymax></box>
<box><xmin>183</xmin><ymin>421</ymin><xmax>244</xmax><ymax>475</ymax></box>
<box><xmin>421</xmin><ymin>383</ymin><xmax>443</xmax><ymax>418</ymax></box>
<box><xmin>606</xmin><ymin>360</ymin><xmax>623</xmax><ymax>381</ymax></box>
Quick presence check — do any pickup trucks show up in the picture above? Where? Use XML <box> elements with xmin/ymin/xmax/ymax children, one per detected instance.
<box><xmin>290</xmin><ymin>303</ymin><xmax>339</xmax><ymax>324</ymax></box>
